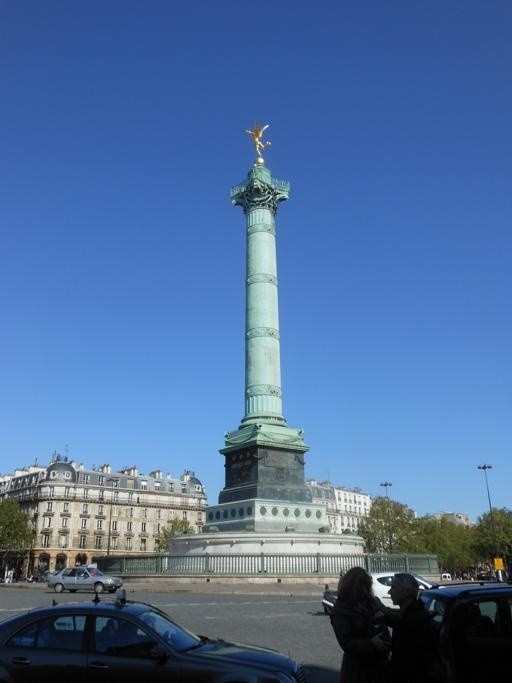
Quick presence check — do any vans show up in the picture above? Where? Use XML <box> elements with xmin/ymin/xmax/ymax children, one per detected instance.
<box><xmin>443</xmin><ymin>574</ymin><xmax>452</xmax><ymax>581</ymax></box>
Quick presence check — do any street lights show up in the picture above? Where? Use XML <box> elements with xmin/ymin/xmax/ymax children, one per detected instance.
<box><xmin>478</xmin><ymin>466</ymin><xmax>497</xmax><ymax>556</ymax></box>
<box><xmin>381</xmin><ymin>482</ymin><xmax>392</xmax><ymax>554</ymax></box>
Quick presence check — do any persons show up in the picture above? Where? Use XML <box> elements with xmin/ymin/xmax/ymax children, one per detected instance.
<box><xmin>374</xmin><ymin>573</ymin><xmax>437</xmax><ymax>683</ymax></box>
<box><xmin>329</xmin><ymin>567</ymin><xmax>391</xmax><ymax>683</ymax></box>
<box><xmin>246</xmin><ymin>124</ymin><xmax>271</xmax><ymax>157</ymax></box>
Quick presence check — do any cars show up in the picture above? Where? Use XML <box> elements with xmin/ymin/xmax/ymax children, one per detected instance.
<box><xmin>322</xmin><ymin>572</ymin><xmax>442</xmax><ymax>615</ymax></box>
<box><xmin>47</xmin><ymin>566</ymin><xmax>122</xmax><ymax>594</ymax></box>
<box><xmin>418</xmin><ymin>585</ymin><xmax>510</xmax><ymax>682</ymax></box>
<box><xmin>1</xmin><ymin>598</ymin><xmax>305</xmax><ymax>683</ymax></box>
